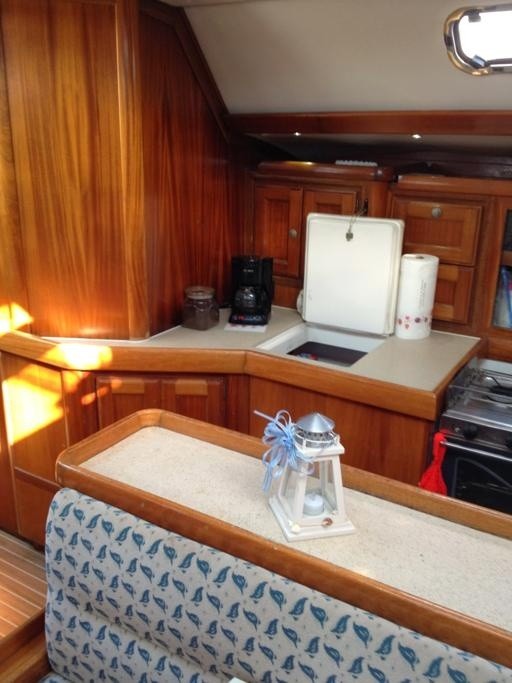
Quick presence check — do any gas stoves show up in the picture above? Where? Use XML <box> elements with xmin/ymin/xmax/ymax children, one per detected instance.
<box><xmin>441</xmin><ymin>353</ymin><xmax>512</xmax><ymax>430</ymax></box>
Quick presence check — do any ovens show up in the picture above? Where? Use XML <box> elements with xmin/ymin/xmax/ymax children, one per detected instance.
<box><xmin>439</xmin><ymin>440</ymin><xmax>512</xmax><ymax>516</ymax></box>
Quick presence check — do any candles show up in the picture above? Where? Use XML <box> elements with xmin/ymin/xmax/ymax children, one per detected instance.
<box><xmin>303</xmin><ymin>489</ymin><xmax>325</xmax><ymax>514</ymax></box>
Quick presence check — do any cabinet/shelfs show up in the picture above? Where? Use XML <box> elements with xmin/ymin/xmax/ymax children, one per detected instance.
<box><xmin>78</xmin><ymin>372</ymin><xmax>250</xmax><ymax>440</ymax></box>
<box><xmin>382</xmin><ymin>174</ymin><xmax>511</xmax><ymax>361</ymax></box>
<box><xmin>237</xmin><ymin>158</ymin><xmax>429</xmax><ymax>334</ymax></box>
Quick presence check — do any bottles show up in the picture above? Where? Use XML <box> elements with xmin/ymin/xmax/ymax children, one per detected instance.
<box><xmin>180</xmin><ymin>286</ymin><xmax>220</xmax><ymax>331</ymax></box>
